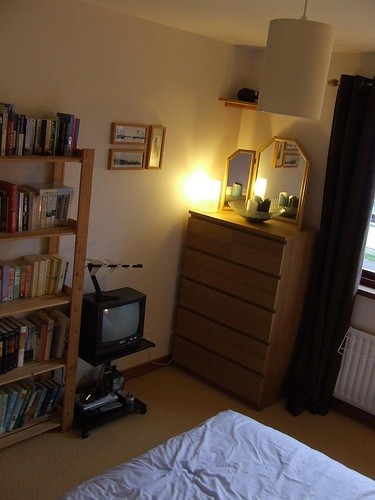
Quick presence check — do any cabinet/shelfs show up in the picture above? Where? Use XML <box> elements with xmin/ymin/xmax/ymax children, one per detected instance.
<box><xmin>0</xmin><ymin>146</ymin><xmax>95</xmax><ymax>449</ymax></box>
<box><xmin>172</xmin><ymin>209</ymin><xmax>319</xmax><ymax>413</ymax></box>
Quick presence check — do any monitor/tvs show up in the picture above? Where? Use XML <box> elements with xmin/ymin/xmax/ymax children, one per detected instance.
<box><xmin>79</xmin><ymin>286</ymin><xmax>146</xmax><ymax>357</ymax></box>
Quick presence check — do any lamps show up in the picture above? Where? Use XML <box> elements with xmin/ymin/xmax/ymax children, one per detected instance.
<box><xmin>255</xmin><ymin>0</ymin><xmax>335</xmax><ymax>120</ymax></box>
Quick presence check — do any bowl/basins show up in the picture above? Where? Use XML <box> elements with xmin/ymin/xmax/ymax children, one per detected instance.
<box><xmin>224</xmin><ymin>187</ymin><xmax>246</xmax><ymax>203</ymax></box>
<box><xmin>271</xmin><ymin>199</ymin><xmax>297</xmax><ymax>218</ymax></box>
<box><xmin>228</xmin><ymin>200</ymin><xmax>287</xmax><ymax>223</ymax></box>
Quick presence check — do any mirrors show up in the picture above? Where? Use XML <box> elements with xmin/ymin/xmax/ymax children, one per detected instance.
<box><xmin>220</xmin><ymin>150</ymin><xmax>256</xmax><ymax>212</ymax></box>
<box><xmin>250</xmin><ymin>136</ymin><xmax>311</xmax><ymax>231</ymax></box>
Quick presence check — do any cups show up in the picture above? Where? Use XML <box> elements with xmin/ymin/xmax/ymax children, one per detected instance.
<box><xmin>247</xmin><ymin>196</ymin><xmax>270</xmax><ymax>212</ymax></box>
<box><xmin>279</xmin><ymin>192</ymin><xmax>299</xmax><ymax>207</ymax></box>
<box><xmin>226</xmin><ymin>182</ymin><xmax>243</xmax><ymax>196</ymax></box>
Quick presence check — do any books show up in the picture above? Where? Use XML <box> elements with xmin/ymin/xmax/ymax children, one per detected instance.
<box><xmin>0</xmin><ymin>378</ymin><xmax>63</xmax><ymax>436</ymax></box>
<box><xmin>0</xmin><ymin>255</ymin><xmax>69</xmax><ymax>303</ymax></box>
<box><xmin>75</xmin><ymin>391</ymin><xmax>118</xmax><ymax>410</ymax></box>
<box><xmin>0</xmin><ymin>180</ymin><xmax>75</xmax><ymax>233</ymax></box>
<box><xmin>0</xmin><ymin>309</ymin><xmax>70</xmax><ymax>376</ymax></box>
<box><xmin>0</xmin><ymin>103</ymin><xmax>80</xmax><ymax>157</ymax></box>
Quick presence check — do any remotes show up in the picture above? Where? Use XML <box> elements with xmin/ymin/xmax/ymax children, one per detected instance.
<box><xmin>99</xmin><ymin>401</ymin><xmax>124</xmax><ymax>413</ymax></box>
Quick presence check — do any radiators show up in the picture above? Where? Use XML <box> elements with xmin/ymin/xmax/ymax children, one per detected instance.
<box><xmin>333</xmin><ymin>328</ymin><xmax>375</xmax><ymax>416</ymax></box>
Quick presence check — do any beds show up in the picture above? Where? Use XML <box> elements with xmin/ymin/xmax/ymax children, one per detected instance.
<box><xmin>64</xmin><ymin>408</ymin><xmax>375</xmax><ymax>500</ymax></box>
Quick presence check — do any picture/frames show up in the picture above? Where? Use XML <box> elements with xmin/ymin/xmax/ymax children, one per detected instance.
<box><xmin>110</xmin><ymin>121</ymin><xmax>149</xmax><ymax>147</ymax></box>
<box><xmin>108</xmin><ymin>147</ymin><xmax>145</xmax><ymax>171</ymax></box>
<box><xmin>143</xmin><ymin>124</ymin><xmax>167</xmax><ymax>170</ymax></box>
